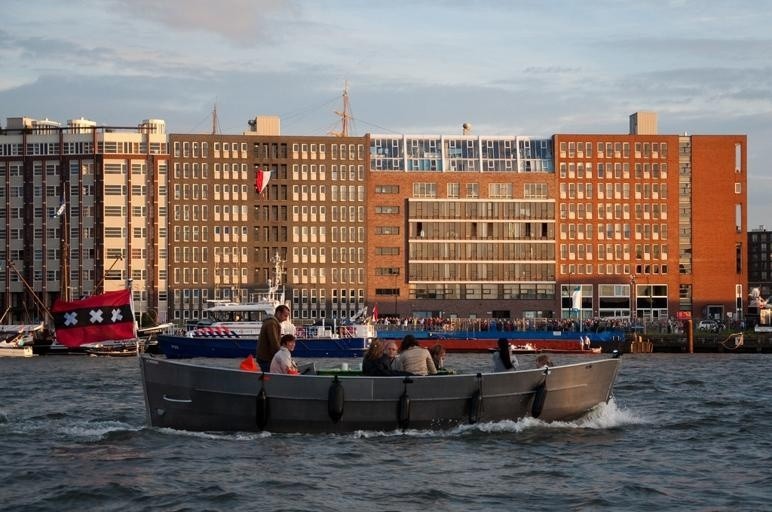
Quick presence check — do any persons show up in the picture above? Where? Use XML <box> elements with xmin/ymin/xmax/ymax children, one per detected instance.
<box><xmin>336</xmin><ymin>316</ymin><xmax>631</xmax><ymax>334</ymax></box>
<box><xmin>576</xmin><ymin>335</ymin><xmax>590</xmax><ymax>350</ymax></box>
<box><xmin>493</xmin><ymin>338</ymin><xmax>519</xmax><ymax>373</ymax></box>
<box><xmin>256</xmin><ymin>305</ymin><xmax>300</xmax><ymax>374</ymax></box>
<box><xmin>362</xmin><ymin>334</ymin><xmax>446</xmax><ymax>375</ymax></box>
<box><xmin>536</xmin><ymin>355</ymin><xmax>553</xmax><ymax>369</ymax></box>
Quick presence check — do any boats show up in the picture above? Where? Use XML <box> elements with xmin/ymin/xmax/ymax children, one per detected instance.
<box><xmin>136</xmin><ymin>352</ymin><xmax>623</xmax><ymax>434</ymax></box>
<box><xmin>0</xmin><ymin>254</ymin><xmax>178</xmax><ymax>358</ymax></box>
<box><xmin>152</xmin><ymin>299</ymin><xmax>378</xmax><ymax>358</ymax></box>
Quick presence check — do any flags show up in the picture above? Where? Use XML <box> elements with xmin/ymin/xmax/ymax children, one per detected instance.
<box><xmin>374</xmin><ymin>304</ymin><xmax>378</xmax><ymax>320</ymax></box>
<box><xmin>572</xmin><ymin>286</ymin><xmax>581</xmax><ymax>311</ymax></box>
<box><xmin>51</xmin><ymin>288</ymin><xmax>137</xmax><ymax>348</ymax></box>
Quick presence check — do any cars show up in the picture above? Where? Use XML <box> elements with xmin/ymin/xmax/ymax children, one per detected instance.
<box><xmin>698</xmin><ymin>318</ymin><xmax>743</xmax><ymax>333</ymax></box>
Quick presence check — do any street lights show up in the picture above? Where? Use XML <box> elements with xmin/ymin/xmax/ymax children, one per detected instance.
<box><xmin>562</xmin><ymin>285</ymin><xmax>572</xmax><ymax>320</ymax></box>
